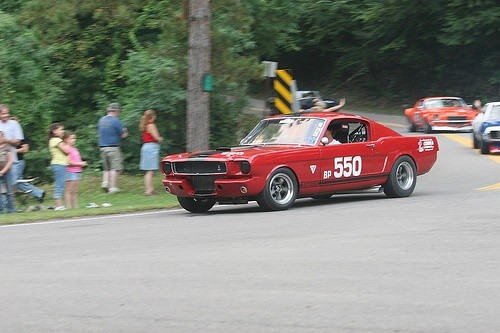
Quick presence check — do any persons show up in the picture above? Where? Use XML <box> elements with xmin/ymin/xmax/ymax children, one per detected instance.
<box><xmin>324</xmin><ymin>126</ymin><xmax>342</xmax><ymax>145</ymax></box>
<box><xmin>98</xmin><ymin>103</ymin><xmax>129</xmax><ymax>194</ymax></box>
<box><xmin>314</xmin><ymin>98</ymin><xmax>345</xmax><ymax>112</ymax></box>
<box><xmin>0</xmin><ymin>104</ymin><xmax>45</xmax><ymax>213</ymax></box>
<box><xmin>139</xmin><ymin>109</ymin><xmax>164</xmax><ymax>195</ymax></box>
<box><xmin>62</xmin><ymin>130</ymin><xmax>88</xmax><ymax>209</ymax></box>
<box><xmin>48</xmin><ymin>122</ymin><xmax>71</xmax><ymax>209</ymax></box>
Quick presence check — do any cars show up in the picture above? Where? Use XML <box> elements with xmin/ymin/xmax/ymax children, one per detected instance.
<box><xmin>161</xmin><ymin>112</ymin><xmax>439</xmax><ymax>213</ymax></box>
<box><xmin>263</xmin><ymin>91</ymin><xmax>337</xmax><ymax>115</ymax></box>
<box><xmin>405</xmin><ymin>97</ymin><xmax>483</xmax><ymax>133</ymax></box>
<box><xmin>471</xmin><ymin>102</ymin><xmax>500</xmax><ymax>153</ymax></box>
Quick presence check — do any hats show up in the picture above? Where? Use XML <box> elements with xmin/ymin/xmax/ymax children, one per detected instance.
<box><xmin>107</xmin><ymin>103</ymin><xmax>122</xmax><ymax>112</ymax></box>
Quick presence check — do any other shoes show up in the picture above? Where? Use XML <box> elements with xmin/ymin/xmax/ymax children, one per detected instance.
<box><xmin>39</xmin><ymin>192</ymin><xmax>46</xmax><ymax>202</ymax></box>
<box><xmin>110</xmin><ymin>188</ymin><xmax>122</xmax><ymax>194</ymax></box>
<box><xmin>102</xmin><ymin>185</ymin><xmax>108</xmax><ymax>191</ymax></box>
<box><xmin>56</xmin><ymin>206</ymin><xmax>66</xmax><ymax>210</ymax></box>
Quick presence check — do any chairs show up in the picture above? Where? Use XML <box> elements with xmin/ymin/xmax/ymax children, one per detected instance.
<box><xmin>11</xmin><ymin>161</ymin><xmax>40</xmax><ymax>204</ymax></box>
